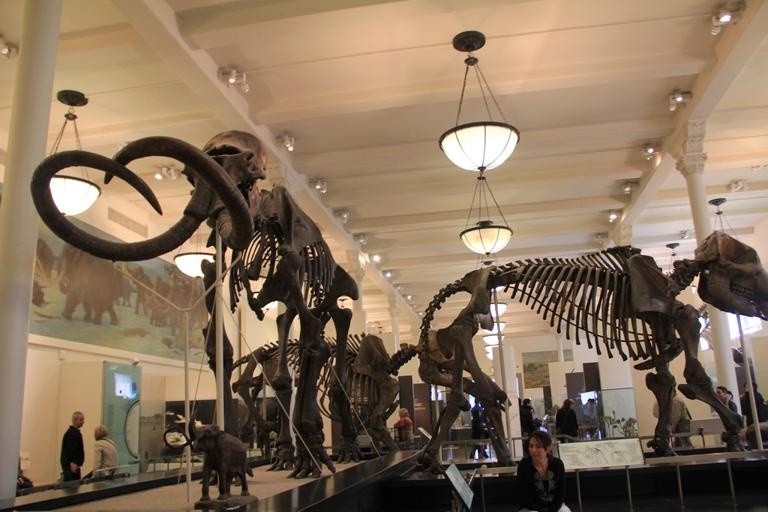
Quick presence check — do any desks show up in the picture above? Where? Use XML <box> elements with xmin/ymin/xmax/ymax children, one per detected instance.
<box><xmin>52</xmin><ymin>434</ymin><xmax>768</xmax><ymax>509</ymax></box>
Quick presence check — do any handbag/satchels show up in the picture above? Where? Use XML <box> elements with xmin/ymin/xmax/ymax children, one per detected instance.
<box><xmin>722</xmin><ymin>432</ymin><xmax>727</xmax><ymax>442</ymax></box>
<box><xmin>676</xmin><ymin>420</ymin><xmax>690</xmax><ymax>433</ymax></box>
<box><xmin>398</xmin><ymin>425</ymin><xmax>408</xmax><ymax>443</ymax></box>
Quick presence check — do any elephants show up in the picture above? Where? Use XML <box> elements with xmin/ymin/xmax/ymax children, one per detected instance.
<box><xmin>187</xmin><ymin>405</ymin><xmax>249</xmax><ymax>501</ymax></box>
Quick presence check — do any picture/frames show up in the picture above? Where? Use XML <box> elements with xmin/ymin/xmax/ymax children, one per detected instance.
<box><xmin>0</xmin><ymin>182</ymin><xmax>209</xmax><ymax>371</ymax></box>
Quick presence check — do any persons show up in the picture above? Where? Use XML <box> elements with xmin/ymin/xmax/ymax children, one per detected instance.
<box><xmin>469</xmin><ymin>398</ymin><xmax>489</xmax><ymax>459</ymax></box>
<box><xmin>60</xmin><ymin>411</ymin><xmax>84</xmax><ymax>481</ymax></box>
<box><xmin>82</xmin><ymin>425</ymin><xmax>120</xmax><ymax>479</ymax></box>
<box><xmin>717</xmin><ymin>382</ymin><xmax>768</xmax><ymax>426</ymax></box>
<box><xmin>394</xmin><ymin>408</ymin><xmax>414</xmax><ymax>444</ymax></box>
<box><xmin>518</xmin><ymin>398</ymin><xmax>599</xmax><ymax>443</ymax></box>
<box><xmin>666</xmin><ymin>388</ymin><xmax>692</xmax><ymax>447</ymax></box>
<box><xmin>516</xmin><ymin>431</ymin><xmax>572</xmax><ymax>512</ymax></box>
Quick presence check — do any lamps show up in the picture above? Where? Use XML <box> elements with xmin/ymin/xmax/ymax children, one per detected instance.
<box><xmin>353</xmin><ymin>233</ymin><xmax>368</xmax><ymax>246</ymax></box>
<box><xmin>309</xmin><ymin>177</ymin><xmax>328</xmax><ymax>194</ymax></box>
<box><xmin>218</xmin><ymin>68</ymin><xmax>249</xmax><ymax>94</ymax></box>
<box><xmin>174</xmin><ymin>190</ymin><xmax>216</xmax><ymax>279</ymax></box>
<box><xmin>253</xmin><ymin>292</ymin><xmax>279</xmax><ymax>311</ymax></box>
<box><xmin>667</xmin><ymin>89</ymin><xmax>693</xmax><ymax>112</ymax></box>
<box><xmin>460</xmin><ymin>172</ymin><xmax>513</xmax><ymax>256</ymax></box>
<box><xmin>478</xmin><ymin>254</ymin><xmax>507</xmax><ymax>362</ymax></box>
<box><xmin>334</xmin><ymin>208</ymin><xmax>350</xmax><ymax>223</ymax></box>
<box><xmin>274</xmin><ymin>131</ymin><xmax>295</xmax><ymax>152</ymax></box>
<box><xmin>438</xmin><ymin>31</ymin><xmax>519</xmax><ymax>176</ymax></box>
<box><xmin>711</xmin><ymin>1</ymin><xmax>745</xmax><ymax>35</ymax></box>
<box><xmin>46</xmin><ymin>90</ymin><xmax>100</xmax><ymax>217</ymax></box>
